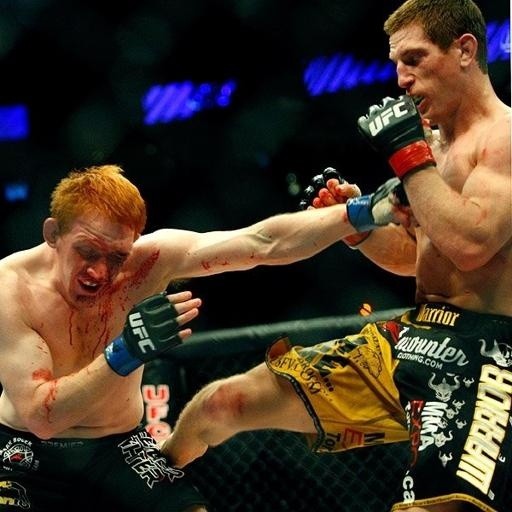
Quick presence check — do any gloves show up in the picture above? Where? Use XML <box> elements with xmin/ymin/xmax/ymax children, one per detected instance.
<box><xmin>298</xmin><ymin>166</ymin><xmax>374</xmax><ymax>251</ymax></box>
<box><xmin>357</xmin><ymin>95</ymin><xmax>437</xmax><ymax>179</ymax></box>
<box><xmin>104</xmin><ymin>294</ymin><xmax>183</xmax><ymax>377</ymax></box>
<box><xmin>346</xmin><ymin>176</ymin><xmax>411</xmax><ymax>234</ymax></box>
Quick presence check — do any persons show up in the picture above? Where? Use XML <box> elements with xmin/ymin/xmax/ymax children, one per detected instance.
<box><xmin>161</xmin><ymin>1</ymin><xmax>511</xmax><ymax>512</ymax></box>
<box><xmin>0</xmin><ymin>157</ymin><xmax>422</xmax><ymax>511</ymax></box>
